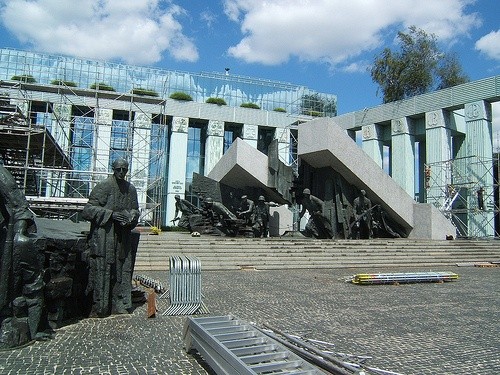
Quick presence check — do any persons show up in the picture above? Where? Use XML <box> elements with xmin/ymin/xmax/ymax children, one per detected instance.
<box><xmin>204</xmin><ymin>197</ymin><xmax>238</xmax><ymax>237</ymax></box>
<box><xmin>174</xmin><ymin>194</ymin><xmax>203</xmax><ymax>234</ymax></box>
<box><xmin>256</xmin><ymin>196</ymin><xmax>269</xmax><ymax>238</ymax></box>
<box><xmin>0</xmin><ymin>162</ymin><xmax>37</xmax><ymax>320</ymax></box>
<box><xmin>81</xmin><ymin>158</ymin><xmax>140</xmax><ymax>318</ymax></box>
<box><xmin>237</xmin><ymin>194</ymin><xmax>257</xmax><ymax>227</ymax></box>
<box><xmin>351</xmin><ymin>189</ymin><xmax>371</xmax><ymax>239</ymax></box>
<box><xmin>297</xmin><ymin>188</ymin><xmax>335</xmax><ymax>240</ymax></box>
